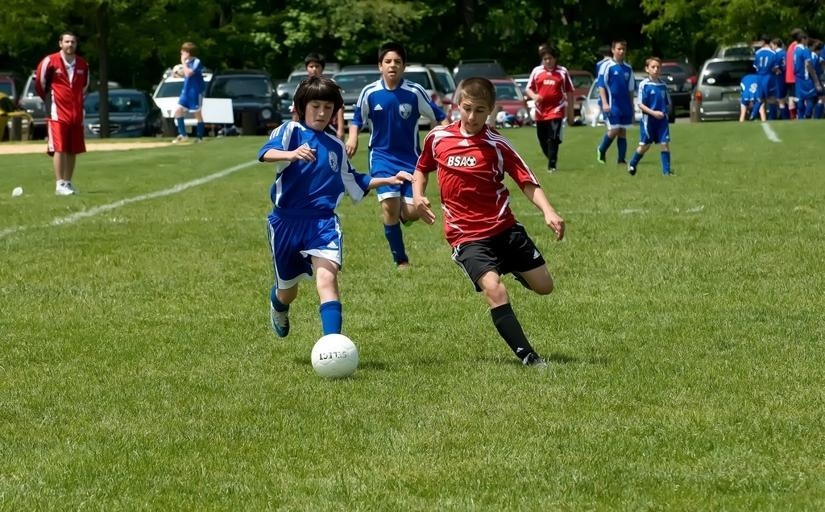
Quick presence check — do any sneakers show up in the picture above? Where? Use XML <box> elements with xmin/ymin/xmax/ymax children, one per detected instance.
<box><xmin>627</xmin><ymin>161</ymin><xmax>637</xmax><ymax>175</ymax></box>
<box><xmin>65</xmin><ymin>180</ymin><xmax>77</xmax><ymax>193</ymax></box>
<box><xmin>270</xmin><ymin>301</ymin><xmax>291</xmax><ymax>338</ymax></box>
<box><xmin>596</xmin><ymin>145</ymin><xmax>606</xmax><ymax>165</ymax></box>
<box><xmin>399</xmin><ymin>214</ymin><xmax>413</xmax><ymax>227</ymax></box>
<box><xmin>55</xmin><ymin>179</ymin><xmax>75</xmax><ymax>195</ymax></box>
<box><xmin>171</xmin><ymin>136</ymin><xmax>189</xmax><ymax>144</ymax></box>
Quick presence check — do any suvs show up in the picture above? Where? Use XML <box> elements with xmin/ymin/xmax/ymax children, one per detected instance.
<box><xmin>278</xmin><ymin>82</ymin><xmax>335</xmax><ymax>123</ymax></box>
<box><xmin>19</xmin><ymin>70</ymin><xmax>52</xmax><ymax>116</ymax></box>
<box><xmin>330</xmin><ymin>70</ymin><xmax>382</xmax><ymax>133</ymax></box>
<box><xmin>153</xmin><ymin>68</ymin><xmax>214</xmax><ymax>135</ymax></box>
<box><xmin>453</xmin><ymin>57</ymin><xmax>507</xmax><ymax>86</ymax></box>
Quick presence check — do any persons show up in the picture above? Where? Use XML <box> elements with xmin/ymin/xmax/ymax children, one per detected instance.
<box><xmin>597</xmin><ymin>39</ymin><xmax>634</xmax><ymax>166</ymax></box>
<box><xmin>345</xmin><ymin>41</ymin><xmax>450</xmax><ymax>270</ymax></box>
<box><xmin>627</xmin><ymin>57</ymin><xmax>673</xmax><ymax>176</ymax></box>
<box><xmin>739</xmin><ymin>28</ymin><xmax>825</xmax><ymax>121</ymax></box>
<box><xmin>172</xmin><ymin>43</ymin><xmax>205</xmax><ymax>144</ymax></box>
<box><xmin>258</xmin><ymin>75</ymin><xmax>416</xmax><ymax>337</ymax></box>
<box><xmin>290</xmin><ymin>53</ymin><xmax>346</xmax><ymax>142</ymax></box>
<box><xmin>35</xmin><ymin>32</ymin><xmax>90</xmax><ymax>196</ymax></box>
<box><xmin>412</xmin><ymin>77</ymin><xmax>566</xmax><ymax>369</ymax></box>
<box><xmin>524</xmin><ymin>47</ymin><xmax>575</xmax><ymax>173</ymax></box>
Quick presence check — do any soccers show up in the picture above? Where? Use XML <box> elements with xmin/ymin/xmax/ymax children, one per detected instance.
<box><xmin>310</xmin><ymin>332</ymin><xmax>360</xmax><ymax>379</ymax></box>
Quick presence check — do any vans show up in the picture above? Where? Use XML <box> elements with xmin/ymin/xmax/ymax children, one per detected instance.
<box><xmin>713</xmin><ymin>39</ymin><xmax>767</xmax><ymax>58</ymax></box>
<box><xmin>288</xmin><ymin>61</ymin><xmax>339</xmax><ymax>81</ymax></box>
<box><xmin>426</xmin><ymin>65</ymin><xmax>458</xmax><ymax>120</ymax></box>
<box><xmin>689</xmin><ymin>56</ymin><xmax>756</xmax><ymax>123</ymax></box>
<box><xmin>402</xmin><ymin>65</ymin><xmax>443</xmax><ymax>130</ymax></box>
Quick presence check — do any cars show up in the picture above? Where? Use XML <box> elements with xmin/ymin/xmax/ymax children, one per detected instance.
<box><xmin>567</xmin><ymin>70</ymin><xmax>594</xmax><ymax>118</ymax></box>
<box><xmin>84</xmin><ymin>89</ymin><xmax>164</xmax><ymax>138</ymax></box>
<box><xmin>582</xmin><ymin>73</ymin><xmax>645</xmax><ymax>126</ymax></box>
<box><xmin>0</xmin><ymin>92</ymin><xmax>34</xmax><ymax>141</ymax></box>
<box><xmin>508</xmin><ymin>73</ymin><xmax>539</xmax><ymax>122</ymax></box>
<box><xmin>443</xmin><ymin>78</ymin><xmax>531</xmax><ymax>128</ymax></box>
<box><xmin>206</xmin><ymin>73</ymin><xmax>281</xmax><ymax>137</ymax></box>
<box><xmin>658</xmin><ymin>62</ymin><xmax>692</xmax><ymax>112</ymax></box>
